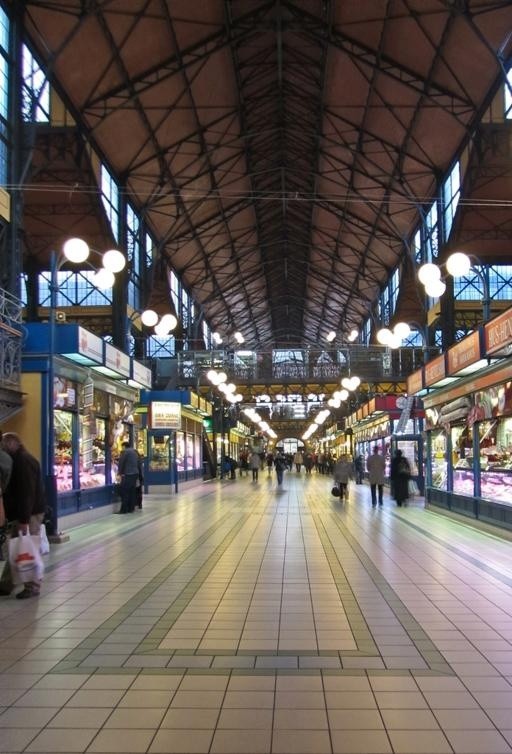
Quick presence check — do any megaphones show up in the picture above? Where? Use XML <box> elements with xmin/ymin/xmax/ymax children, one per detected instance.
<box><xmin>56</xmin><ymin>311</ymin><xmax>66</xmax><ymax>322</ymax></box>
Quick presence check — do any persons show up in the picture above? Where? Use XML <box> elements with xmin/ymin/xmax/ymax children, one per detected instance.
<box><xmin>0</xmin><ymin>449</ymin><xmax>16</xmax><ymax>596</ymax></box>
<box><xmin>131</xmin><ymin>449</ymin><xmax>145</xmax><ymax>511</ymax></box>
<box><xmin>5</xmin><ymin>431</ymin><xmax>48</xmax><ymax>599</ymax></box>
<box><xmin>221</xmin><ymin>444</ymin><xmax>411</xmax><ymax>508</ymax></box>
<box><xmin>114</xmin><ymin>441</ymin><xmax>140</xmax><ymax>513</ymax></box>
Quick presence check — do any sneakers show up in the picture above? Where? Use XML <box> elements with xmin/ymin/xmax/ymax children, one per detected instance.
<box><xmin>0</xmin><ymin>586</ymin><xmax>42</xmax><ymax>599</ymax></box>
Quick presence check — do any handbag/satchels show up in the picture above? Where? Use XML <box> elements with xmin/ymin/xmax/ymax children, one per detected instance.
<box><xmin>7</xmin><ymin>524</ymin><xmax>49</xmax><ymax>589</ymax></box>
<box><xmin>407</xmin><ymin>479</ymin><xmax>421</xmax><ymax>496</ymax></box>
<box><xmin>331</xmin><ymin>487</ymin><xmax>339</xmax><ymax>497</ymax></box>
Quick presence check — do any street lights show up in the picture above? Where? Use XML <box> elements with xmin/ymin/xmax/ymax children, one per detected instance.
<box><xmin>43</xmin><ymin>238</ymin><xmax>127</xmax><ymax>544</ymax></box>
